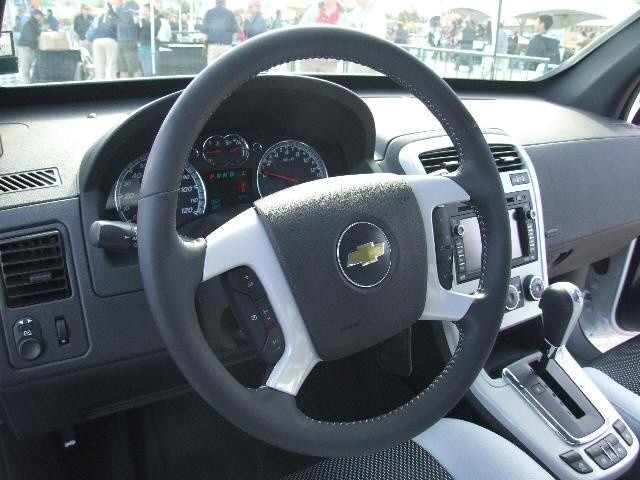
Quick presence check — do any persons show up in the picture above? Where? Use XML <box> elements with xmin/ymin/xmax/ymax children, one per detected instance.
<box><xmin>13</xmin><ymin>1</ymin><xmax>595</xmax><ymax>82</ymax></box>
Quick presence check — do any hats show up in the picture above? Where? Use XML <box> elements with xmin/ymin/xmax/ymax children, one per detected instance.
<box><xmin>32</xmin><ymin>9</ymin><xmax>45</xmax><ymax>15</ymax></box>
